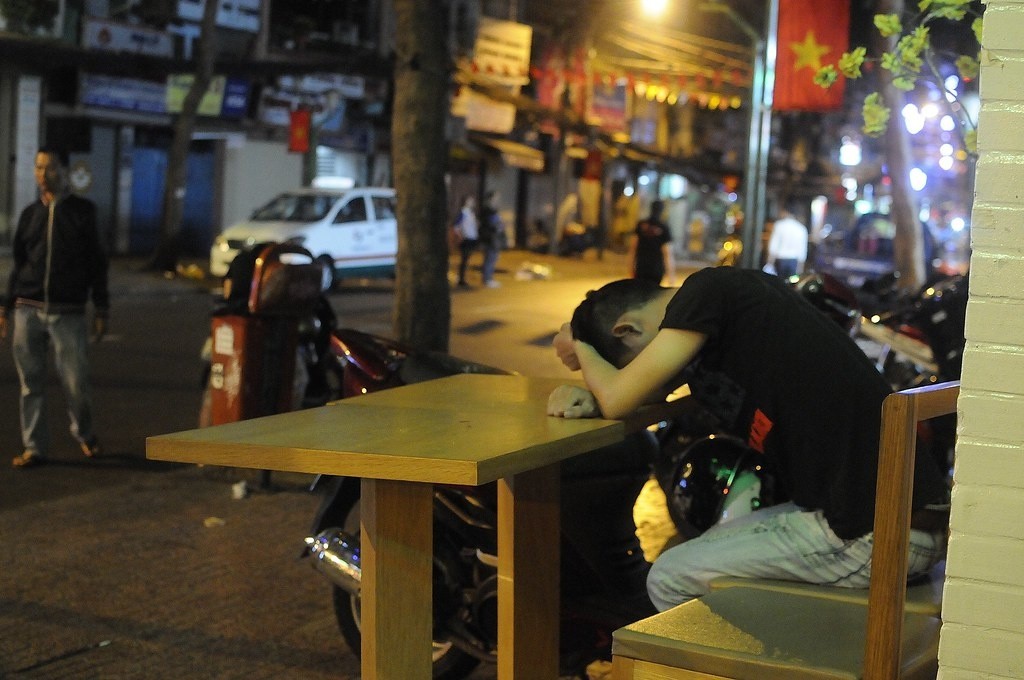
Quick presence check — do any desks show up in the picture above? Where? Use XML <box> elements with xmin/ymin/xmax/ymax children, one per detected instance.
<box><xmin>145</xmin><ymin>369</ymin><xmax>696</xmax><ymax>680</ymax></box>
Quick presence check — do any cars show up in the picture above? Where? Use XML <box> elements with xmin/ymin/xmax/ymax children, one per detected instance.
<box><xmin>208</xmin><ymin>175</ymin><xmax>398</xmax><ymax>294</ymax></box>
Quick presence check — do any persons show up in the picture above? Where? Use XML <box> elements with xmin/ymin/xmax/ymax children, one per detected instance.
<box><xmin>482</xmin><ymin>191</ymin><xmax>506</xmax><ymax>287</ymax></box>
<box><xmin>454</xmin><ymin>195</ymin><xmax>480</xmax><ymax>290</ymax></box>
<box><xmin>554</xmin><ymin>267</ymin><xmax>949</xmax><ymax>615</ymax></box>
<box><xmin>628</xmin><ymin>201</ymin><xmax>675</xmax><ymax>287</ymax></box>
<box><xmin>719</xmin><ymin>206</ymin><xmax>809</xmax><ymax>282</ymax></box>
<box><xmin>1</xmin><ymin>147</ymin><xmax>109</xmax><ymax>466</ymax></box>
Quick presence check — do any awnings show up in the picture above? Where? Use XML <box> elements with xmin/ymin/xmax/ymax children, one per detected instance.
<box><xmin>470</xmin><ymin>134</ymin><xmax>544</xmax><ymax>173</ymax></box>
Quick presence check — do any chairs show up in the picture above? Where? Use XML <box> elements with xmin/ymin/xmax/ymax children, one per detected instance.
<box><xmin>608</xmin><ymin>377</ymin><xmax>962</xmax><ymax>680</ymax></box>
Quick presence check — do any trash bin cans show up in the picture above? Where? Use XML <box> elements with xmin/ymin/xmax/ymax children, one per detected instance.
<box><xmin>199</xmin><ymin>242</ymin><xmax>324</xmax><ymax>493</ymax></box>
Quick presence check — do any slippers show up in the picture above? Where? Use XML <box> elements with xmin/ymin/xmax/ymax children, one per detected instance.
<box><xmin>13</xmin><ymin>447</ymin><xmax>45</xmax><ymax>466</ymax></box>
<box><xmin>80</xmin><ymin>437</ymin><xmax>100</xmax><ymax>456</ymax></box>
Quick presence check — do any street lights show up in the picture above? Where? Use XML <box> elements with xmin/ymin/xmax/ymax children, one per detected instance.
<box><xmin>638</xmin><ymin>0</ymin><xmax>767</xmax><ymax>269</ymax></box>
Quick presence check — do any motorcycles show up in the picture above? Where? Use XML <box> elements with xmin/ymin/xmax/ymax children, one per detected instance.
<box><xmin>302</xmin><ymin>269</ymin><xmax>856</xmax><ymax>680</ymax></box>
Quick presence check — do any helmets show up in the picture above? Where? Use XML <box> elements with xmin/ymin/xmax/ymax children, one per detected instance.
<box><xmin>665</xmin><ymin>431</ymin><xmax>768</xmax><ymax>539</ymax></box>
<box><xmin>793</xmin><ymin>269</ymin><xmax>860</xmax><ymax>340</ymax></box>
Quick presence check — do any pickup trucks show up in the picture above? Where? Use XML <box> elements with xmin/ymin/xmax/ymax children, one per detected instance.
<box><xmin>832</xmin><ymin>211</ymin><xmax>936</xmax><ymax>293</ymax></box>
